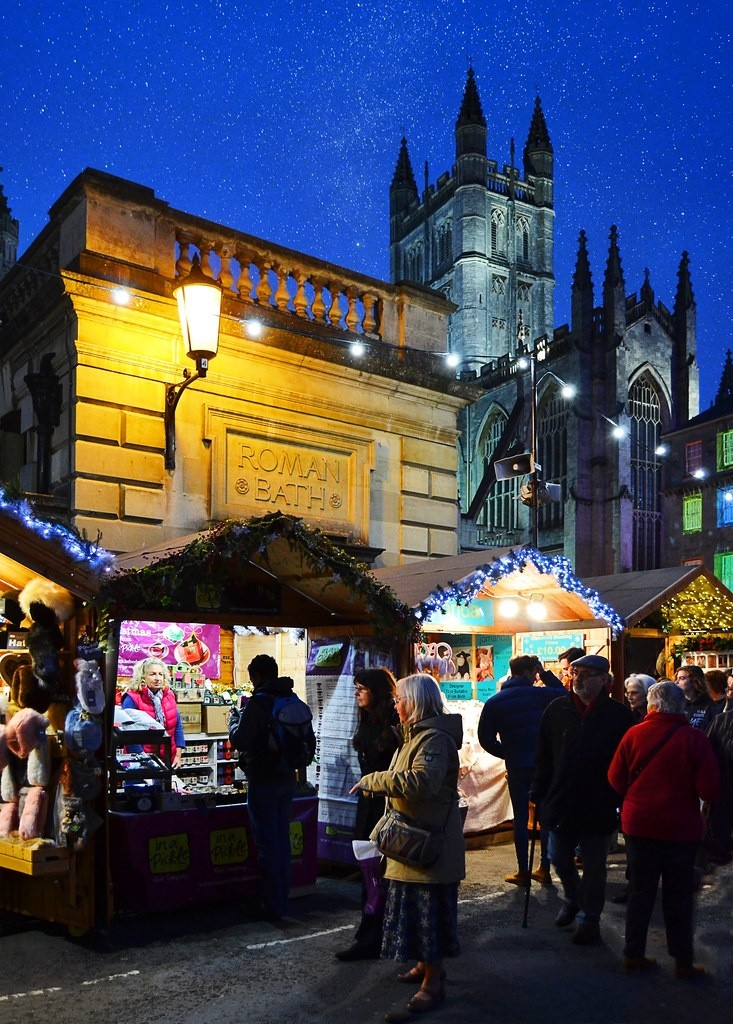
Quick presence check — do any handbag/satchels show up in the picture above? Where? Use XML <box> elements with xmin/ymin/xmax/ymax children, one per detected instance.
<box><xmin>352</xmin><ymin>838</ymin><xmax>389</xmax><ymax>919</ymax></box>
<box><xmin>375</xmin><ymin>809</ymin><xmax>441</xmax><ymax>870</ymax></box>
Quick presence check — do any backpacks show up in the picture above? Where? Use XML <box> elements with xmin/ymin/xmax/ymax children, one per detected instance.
<box><xmin>247</xmin><ymin>692</ymin><xmax>316</xmax><ymax>770</ymax></box>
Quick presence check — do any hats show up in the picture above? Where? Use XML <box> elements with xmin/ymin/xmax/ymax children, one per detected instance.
<box><xmin>0</xmin><ymin>576</ymin><xmax>106</xmax><ymax>846</ymax></box>
<box><xmin>248</xmin><ymin>654</ymin><xmax>277</xmax><ymax>678</ymax></box>
<box><xmin>570</xmin><ymin>655</ymin><xmax>610</xmax><ymax>674</ymax></box>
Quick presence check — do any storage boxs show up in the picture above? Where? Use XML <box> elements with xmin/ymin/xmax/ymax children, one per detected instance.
<box><xmin>176</xmin><ymin>703</ymin><xmax>201</xmax><ymax>733</ymax></box>
<box><xmin>202</xmin><ymin>706</ymin><xmax>232</xmax><ymax>733</ymax></box>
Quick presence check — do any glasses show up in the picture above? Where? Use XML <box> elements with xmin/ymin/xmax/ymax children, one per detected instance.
<box><xmin>569</xmin><ymin>671</ymin><xmax>602</xmax><ymax>680</ymax></box>
<box><xmin>354</xmin><ymin>686</ymin><xmax>370</xmax><ymax>694</ymax></box>
<box><xmin>393</xmin><ymin>697</ymin><xmax>408</xmax><ymax>704</ymax></box>
<box><xmin>725</xmin><ymin>686</ymin><xmax>732</xmax><ymax>693</ymax></box>
<box><xmin>624</xmin><ymin>691</ymin><xmax>641</xmax><ymax>697</ymax></box>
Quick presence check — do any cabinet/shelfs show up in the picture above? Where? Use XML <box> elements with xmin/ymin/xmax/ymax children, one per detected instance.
<box><xmin>168</xmin><ymin>734</ymin><xmax>247</xmax><ymax>794</ymax></box>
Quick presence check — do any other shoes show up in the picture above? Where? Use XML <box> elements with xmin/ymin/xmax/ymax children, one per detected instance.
<box><xmin>240</xmin><ymin>899</ymin><xmax>280</xmax><ymax>922</ymax></box>
<box><xmin>333</xmin><ymin>937</ymin><xmax>382</xmax><ymax>959</ymax></box>
<box><xmin>555</xmin><ymin>896</ymin><xmax>581</xmax><ymax>927</ymax></box>
<box><xmin>572</xmin><ymin>852</ymin><xmax>584</xmax><ymax>867</ymax></box>
<box><xmin>608</xmin><ymin>842</ymin><xmax>620</xmax><ymax>854</ymax></box>
<box><xmin>406</xmin><ymin>982</ymin><xmax>445</xmax><ymax>1012</ymax></box>
<box><xmin>397</xmin><ymin>962</ymin><xmax>446</xmax><ymax>982</ymax></box>
<box><xmin>571</xmin><ymin>920</ymin><xmax>600</xmax><ymax>944</ymax></box>
<box><xmin>613</xmin><ymin>886</ymin><xmax>629</xmax><ymax>903</ymax></box>
<box><xmin>675</xmin><ymin>960</ymin><xmax>704</xmax><ymax>976</ymax></box>
<box><xmin>505</xmin><ymin>870</ymin><xmax>531</xmax><ymax>887</ymax></box>
<box><xmin>531</xmin><ymin>867</ymin><xmax>552</xmax><ymax>883</ymax></box>
<box><xmin>624</xmin><ymin>953</ymin><xmax>656</xmax><ymax>974</ymax></box>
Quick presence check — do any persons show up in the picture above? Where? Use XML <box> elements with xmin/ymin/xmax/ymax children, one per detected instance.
<box><xmin>228</xmin><ymin>654</ymin><xmax>318</xmax><ymax>917</ymax></box>
<box><xmin>335</xmin><ymin>668</ymin><xmax>403</xmax><ymax>963</ymax></box>
<box><xmin>120</xmin><ymin>657</ymin><xmax>188</xmax><ymax>774</ymax></box>
<box><xmin>349</xmin><ymin>673</ymin><xmax>466</xmax><ymax>1013</ymax></box>
<box><xmin>477</xmin><ymin>648</ymin><xmax>733</xmax><ymax>982</ymax></box>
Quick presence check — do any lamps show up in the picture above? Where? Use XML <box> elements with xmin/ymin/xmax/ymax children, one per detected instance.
<box><xmin>163</xmin><ymin>252</ymin><xmax>225</xmax><ymax>470</ymax></box>
